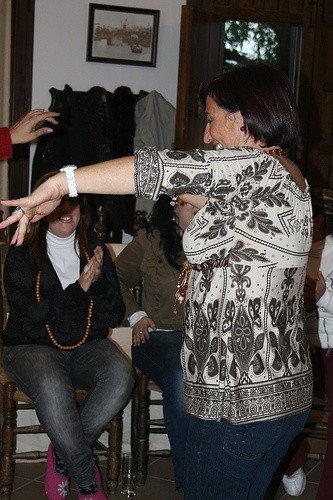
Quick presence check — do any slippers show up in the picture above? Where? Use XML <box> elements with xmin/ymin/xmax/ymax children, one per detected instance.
<box><xmin>75</xmin><ymin>465</ymin><xmax>108</xmax><ymax>500</ymax></box>
<box><xmin>44</xmin><ymin>440</ymin><xmax>70</xmax><ymax>500</ymax></box>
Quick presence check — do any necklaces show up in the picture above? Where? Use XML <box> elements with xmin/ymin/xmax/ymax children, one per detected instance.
<box><xmin>171</xmin><ymin>148</ymin><xmax>285</xmax><ymax>316</ymax></box>
<box><xmin>36</xmin><ymin>243</ymin><xmax>95</xmax><ymax>349</ymax></box>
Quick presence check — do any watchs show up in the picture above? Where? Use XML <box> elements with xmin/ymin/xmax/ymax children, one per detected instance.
<box><xmin>59</xmin><ymin>166</ymin><xmax>78</xmax><ymax>197</ymax></box>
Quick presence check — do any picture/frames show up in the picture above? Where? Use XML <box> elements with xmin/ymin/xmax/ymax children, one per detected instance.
<box><xmin>85</xmin><ymin>3</ymin><xmax>160</xmax><ymax>68</ymax></box>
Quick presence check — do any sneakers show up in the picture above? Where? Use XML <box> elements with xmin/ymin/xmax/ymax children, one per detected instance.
<box><xmin>282</xmin><ymin>465</ymin><xmax>306</xmax><ymax>496</ymax></box>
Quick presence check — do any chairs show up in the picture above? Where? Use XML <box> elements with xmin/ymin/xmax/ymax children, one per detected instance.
<box><xmin>0</xmin><ymin>240</ymin><xmax>123</xmax><ymax>500</ymax></box>
<box><xmin>131</xmin><ymin>282</ymin><xmax>171</xmax><ymax>486</ymax></box>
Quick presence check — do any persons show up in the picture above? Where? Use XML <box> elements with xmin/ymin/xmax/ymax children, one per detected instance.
<box><xmin>0</xmin><ymin>171</ymin><xmax>135</xmax><ymax>500</ymax></box>
<box><xmin>0</xmin><ymin>63</ymin><xmax>317</xmax><ymax>500</ymax></box>
<box><xmin>113</xmin><ymin>192</ymin><xmax>333</xmax><ymax>496</ymax></box>
<box><xmin>0</xmin><ymin>108</ymin><xmax>60</xmax><ymax>161</ymax></box>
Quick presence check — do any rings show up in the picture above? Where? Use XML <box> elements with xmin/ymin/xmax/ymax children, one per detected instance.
<box><xmin>138</xmin><ymin>331</ymin><xmax>142</xmax><ymax>334</ymax></box>
<box><xmin>19</xmin><ymin>209</ymin><xmax>25</xmax><ymax>215</ymax></box>
<box><xmin>97</xmin><ymin>264</ymin><xmax>101</xmax><ymax>270</ymax></box>
